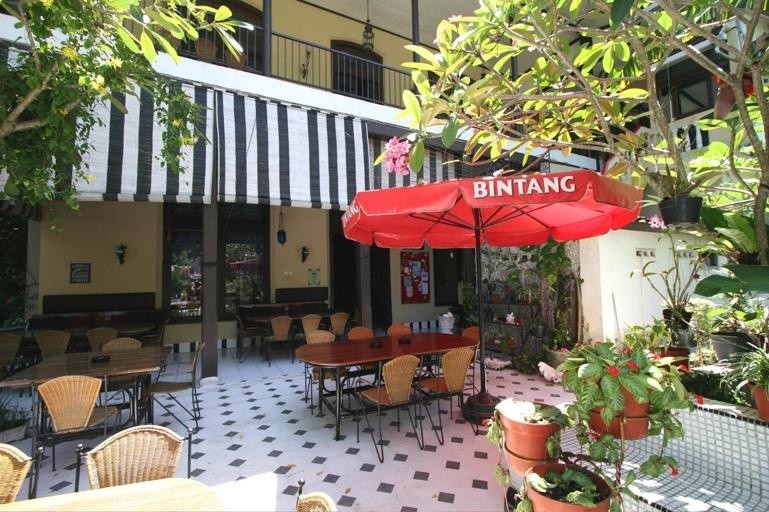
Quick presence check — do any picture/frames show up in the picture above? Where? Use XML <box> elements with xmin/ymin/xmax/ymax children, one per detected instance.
<box><xmin>70</xmin><ymin>262</ymin><xmax>91</xmax><ymax>283</ymax></box>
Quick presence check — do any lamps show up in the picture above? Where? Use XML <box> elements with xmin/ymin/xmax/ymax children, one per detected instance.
<box><xmin>115</xmin><ymin>243</ymin><xmax>127</xmax><ymax>266</ymax></box>
<box><xmin>277</xmin><ymin>205</ymin><xmax>287</xmax><ymax>246</ymax></box>
<box><xmin>301</xmin><ymin>245</ymin><xmax>311</xmax><ymax>262</ymax></box>
<box><xmin>361</xmin><ymin>0</ymin><xmax>374</xmax><ymax>55</ymax></box>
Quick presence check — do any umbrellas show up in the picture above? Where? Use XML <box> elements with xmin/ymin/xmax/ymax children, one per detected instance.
<box><xmin>341</xmin><ymin>169</ymin><xmax>644</xmax><ymax>395</ymax></box>
